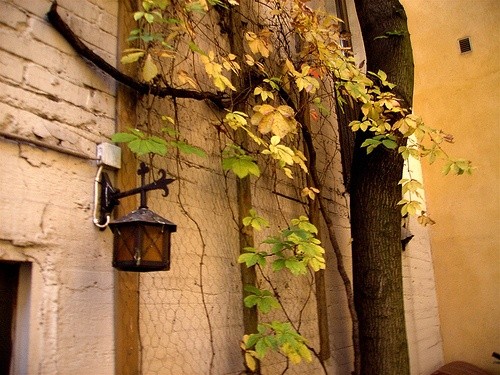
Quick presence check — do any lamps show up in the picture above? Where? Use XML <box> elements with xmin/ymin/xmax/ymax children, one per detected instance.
<box><xmin>98</xmin><ymin>160</ymin><xmax>177</xmax><ymax>276</ymax></box>
